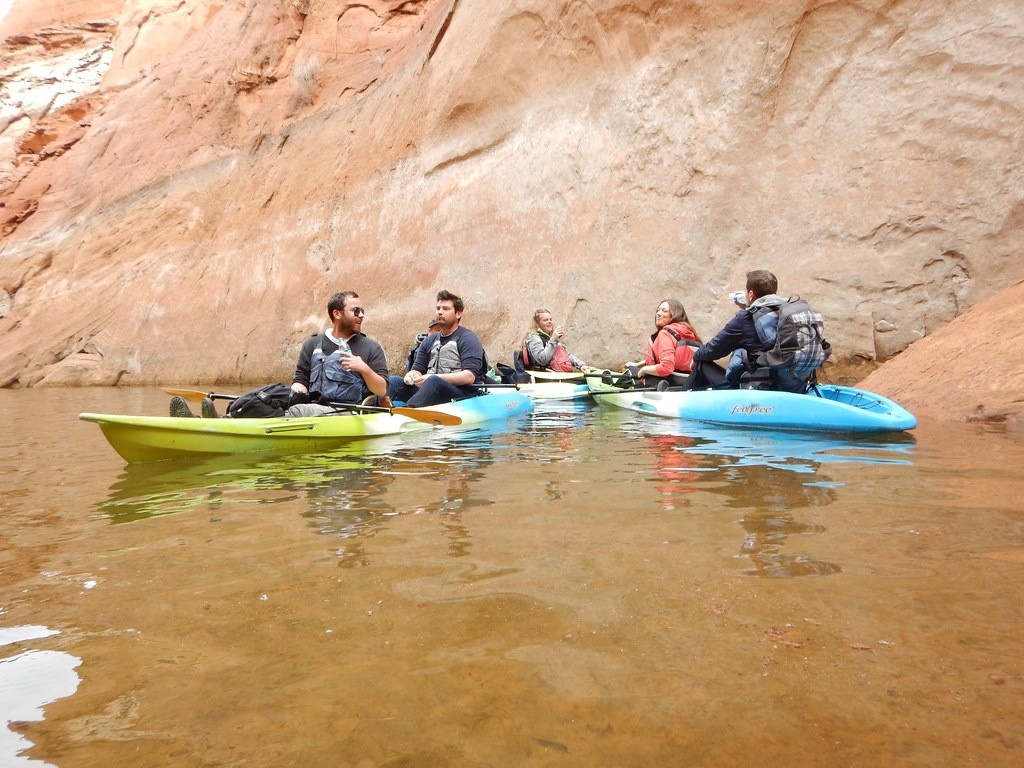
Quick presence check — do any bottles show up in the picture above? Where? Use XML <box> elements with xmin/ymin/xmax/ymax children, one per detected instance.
<box><xmin>728</xmin><ymin>292</ymin><xmax>746</xmax><ymax>304</ymax></box>
<box><xmin>338</xmin><ymin>337</ymin><xmax>353</xmax><ymax>371</ymax></box>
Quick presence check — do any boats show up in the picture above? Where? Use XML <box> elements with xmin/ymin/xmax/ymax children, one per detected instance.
<box><xmin>79</xmin><ymin>390</ymin><xmax>539</xmax><ymax>465</ymax></box>
<box><xmin>520</xmin><ymin>372</ymin><xmax>588</xmax><ymax>401</ymax></box>
<box><xmin>589</xmin><ymin>364</ymin><xmax>917</xmax><ymax>433</ymax></box>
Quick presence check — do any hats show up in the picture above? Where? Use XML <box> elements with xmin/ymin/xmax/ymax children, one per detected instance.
<box><xmin>428</xmin><ymin>319</ymin><xmax>438</xmax><ymax>328</ymax></box>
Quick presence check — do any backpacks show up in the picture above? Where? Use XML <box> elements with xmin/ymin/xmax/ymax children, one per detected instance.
<box><xmin>750</xmin><ymin>294</ymin><xmax>832</xmax><ymax>393</ymax></box>
<box><xmin>229</xmin><ymin>381</ymin><xmax>329</xmax><ymax>418</ymax></box>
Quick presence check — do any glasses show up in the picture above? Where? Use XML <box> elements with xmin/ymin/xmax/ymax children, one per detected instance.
<box><xmin>335</xmin><ymin>307</ymin><xmax>364</xmax><ymax>317</ymax></box>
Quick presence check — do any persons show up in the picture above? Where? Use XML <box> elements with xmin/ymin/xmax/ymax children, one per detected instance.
<box><xmin>525</xmin><ymin>309</ymin><xmax>595</xmax><ymax>385</ymax></box>
<box><xmin>169</xmin><ymin>291</ymin><xmax>390</xmax><ymax>418</ymax></box>
<box><xmin>658</xmin><ymin>269</ymin><xmax>789</xmax><ymax>392</ymax></box>
<box><xmin>602</xmin><ymin>299</ymin><xmax>703</xmax><ymax>388</ymax></box>
<box><xmin>371</xmin><ymin>290</ymin><xmax>484</xmax><ymax>413</ymax></box>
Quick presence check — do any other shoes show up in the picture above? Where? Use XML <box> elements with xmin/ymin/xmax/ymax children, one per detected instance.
<box><xmin>380</xmin><ymin>396</ymin><xmax>394</xmax><ymax>411</ymax></box>
<box><xmin>657</xmin><ymin>380</ymin><xmax>672</xmax><ymax>393</ymax></box>
<box><xmin>602</xmin><ymin>371</ymin><xmax>613</xmax><ymax>387</ymax></box>
<box><xmin>169</xmin><ymin>396</ymin><xmax>193</xmax><ymax>417</ymax></box>
<box><xmin>201</xmin><ymin>398</ymin><xmax>219</xmax><ymax>418</ymax></box>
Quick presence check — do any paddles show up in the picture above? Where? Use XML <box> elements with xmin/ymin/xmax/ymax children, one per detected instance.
<box><xmin>159</xmin><ymin>386</ymin><xmax>464</xmax><ymax>427</ymax></box>
<box><xmin>519</xmin><ymin>382</ymin><xmax>712</xmax><ymax>402</ymax></box>
<box><xmin>459</xmin><ymin>381</ymin><xmax>580</xmax><ymax>400</ymax></box>
<box><xmin>523</xmin><ymin>369</ymin><xmax>641</xmax><ymax>382</ymax></box>
